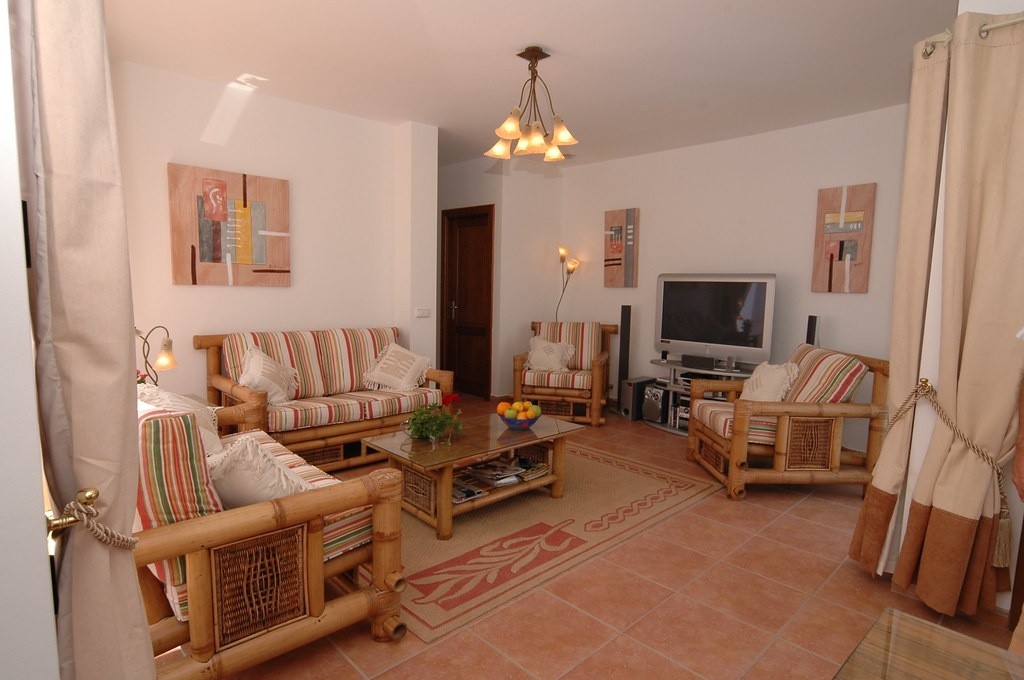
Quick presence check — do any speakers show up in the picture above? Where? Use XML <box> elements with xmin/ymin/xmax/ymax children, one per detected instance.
<box><xmin>617</xmin><ymin>305</ymin><xmax>631</xmax><ymax>411</ymax></box>
<box><xmin>807</xmin><ymin>315</ymin><xmax>820</xmax><ymax>347</ymax></box>
<box><xmin>621</xmin><ymin>376</ymin><xmax>657</xmax><ymax>421</ymax></box>
<box><xmin>680</xmin><ymin>354</ymin><xmax>715</xmax><ymax>370</ymax></box>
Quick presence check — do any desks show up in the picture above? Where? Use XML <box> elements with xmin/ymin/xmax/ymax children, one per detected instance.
<box><xmin>832</xmin><ymin>607</ymin><xmax>1024</xmax><ymax>680</ymax></box>
<box><xmin>644</xmin><ymin>356</ymin><xmax>751</xmax><ymax>438</ymax></box>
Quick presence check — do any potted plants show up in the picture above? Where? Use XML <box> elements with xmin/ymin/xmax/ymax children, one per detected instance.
<box><xmin>401</xmin><ymin>404</ymin><xmax>463</xmax><ymax>449</ymax></box>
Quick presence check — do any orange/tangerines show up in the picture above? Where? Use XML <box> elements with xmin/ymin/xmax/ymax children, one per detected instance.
<box><xmin>526</xmin><ymin>410</ymin><xmax>535</xmax><ymax>419</ymax></box>
<box><xmin>523</xmin><ymin>401</ymin><xmax>533</xmax><ymax>410</ymax></box>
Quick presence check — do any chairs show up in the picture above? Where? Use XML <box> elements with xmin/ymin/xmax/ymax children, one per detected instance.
<box><xmin>688</xmin><ymin>344</ymin><xmax>890</xmax><ymax>500</ymax></box>
<box><xmin>512</xmin><ymin>321</ymin><xmax>618</xmax><ymax>428</ymax></box>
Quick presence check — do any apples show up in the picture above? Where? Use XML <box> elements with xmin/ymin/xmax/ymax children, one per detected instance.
<box><xmin>497</xmin><ymin>401</ymin><xmax>541</xmax><ymax>420</ymax></box>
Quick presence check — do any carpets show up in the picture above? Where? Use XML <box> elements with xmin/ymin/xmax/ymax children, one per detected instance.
<box><xmin>326</xmin><ymin>440</ymin><xmax>728</xmax><ymax>643</ymax></box>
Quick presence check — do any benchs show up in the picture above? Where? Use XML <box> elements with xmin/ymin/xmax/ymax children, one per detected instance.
<box><xmin>130</xmin><ymin>402</ymin><xmax>407</xmax><ymax>680</ymax></box>
<box><xmin>193</xmin><ymin>326</ymin><xmax>454</xmax><ymax>472</ymax></box>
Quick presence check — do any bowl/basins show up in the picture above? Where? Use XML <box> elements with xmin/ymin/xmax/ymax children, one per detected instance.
<box><xmin>500</xmin><ymin>417</ymin><xmax>540</xmax><ymax>431</ymax></box>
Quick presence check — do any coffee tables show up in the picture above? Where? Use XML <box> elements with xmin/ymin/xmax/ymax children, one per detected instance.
<box><xmin>360</xmin><ymin>410</ymin><xmax>588</xmax><ymax>540</ymax></box>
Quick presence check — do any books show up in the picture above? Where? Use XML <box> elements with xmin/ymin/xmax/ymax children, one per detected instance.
<box><xmin>452</xmin><ymin>451</ymin><xmax>552</xmax><ymax>504</ymax></box>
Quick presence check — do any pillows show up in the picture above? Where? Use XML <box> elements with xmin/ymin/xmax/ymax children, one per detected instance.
<box><xmin>367</xmin><ymin>343</ymin><xmax>431</xmax><ymax>394</ymax></box>
<box><xmin>216</xmin><ymin>440</ymin><xmax>310</xmax><ymax>506</ymax></box>
<box><xmin>740</xmin><ymin>362</ymin><xmax>793</xmax><ymax>403</ymax></box>
<box><xmin>243</xmin><ymin>346</ymin><xmax>295</xmax><ymax>402</ymax></box>
<box><xmin>527</xmin><ymin>338</ymin><xmax>574</xmax><ymax>372</ymax></box>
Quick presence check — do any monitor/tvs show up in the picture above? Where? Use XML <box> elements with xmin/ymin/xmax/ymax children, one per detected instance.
<box><xmin>654</xmin><ymin>273</ymin><xmax>776</xmax><ymax>371</ymax></box>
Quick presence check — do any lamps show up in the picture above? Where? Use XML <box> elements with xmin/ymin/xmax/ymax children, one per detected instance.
<box><xmin>484</xmin><ymin>47</ymin><xmax>579</xmax><ymax>163</ymax></box>
<box><xmin>554</xmin><ymin>246</ymin><xmax>579</xmax><ymax>322</ymax></box>
<box><xmin>135</xmin><ymin>326</ymin><xmax>176</xmax><ymax>384</ymax></box>
